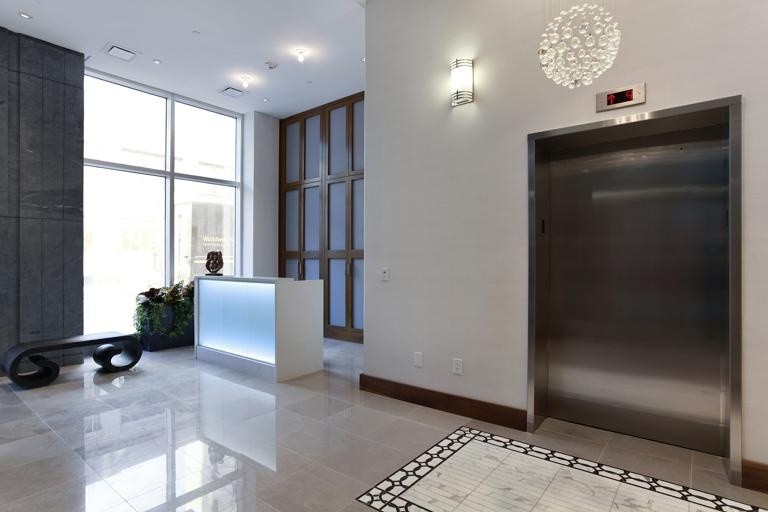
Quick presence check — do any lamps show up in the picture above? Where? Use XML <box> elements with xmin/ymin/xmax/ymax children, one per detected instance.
<box><xmin>537</xmin><ymin>1</ymin><xmax>622</xmax><ymax>89</ymax></box>
<box><xmin>450</xmin><ymin>58</ymin><xmax>474</xmax><ymax>107</ymax></box>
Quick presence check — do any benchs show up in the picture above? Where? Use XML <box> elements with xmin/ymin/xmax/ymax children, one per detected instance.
<box><xmin>2</xmin><ymin>331</ymin><xmax>143</xmax><ymax>389</ymax></box>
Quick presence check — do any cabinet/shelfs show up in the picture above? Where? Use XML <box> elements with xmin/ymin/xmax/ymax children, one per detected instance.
<box><xmin>0</xmin><ymin>28</ymin><xmax>84</xmax><ymax>376</ymax></box>
<box><xmin>280</xmin><ymin>91</ymin><xmax>364</xmax><ymax>345</ymax></box>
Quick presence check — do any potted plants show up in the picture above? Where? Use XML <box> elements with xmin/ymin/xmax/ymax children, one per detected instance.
<box><xmin>135</xmin><ymin>280</ymin><xmax>194</xmax><ymax>352</ymax></box>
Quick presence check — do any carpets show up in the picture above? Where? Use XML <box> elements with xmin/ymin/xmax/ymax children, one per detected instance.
<box><xmin>355</xmin><ymin>426</ymin><xmax>767</xmax><ymax>512</ymax></box>
